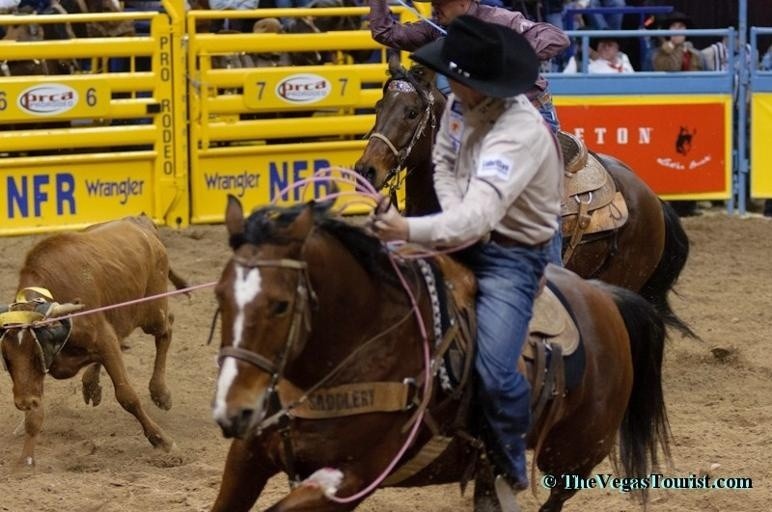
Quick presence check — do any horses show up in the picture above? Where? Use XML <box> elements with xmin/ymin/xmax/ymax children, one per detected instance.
<box><xmin>211</xmin><ymin>193</ymin><xmax>676</xmax><ymax>512</ymax></box>
<box><xmin>355</xmin><ymin>50</ymin><xmax>702</xmax><ymax>342</ymax></box>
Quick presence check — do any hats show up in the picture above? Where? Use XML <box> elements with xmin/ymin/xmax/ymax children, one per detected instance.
<box><xmin>407</xmin><ymin>14</ymin><xmax>540</xmax><ymax>100</ymax></box>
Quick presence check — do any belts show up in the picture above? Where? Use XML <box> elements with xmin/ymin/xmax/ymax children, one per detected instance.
<box><xmin>529</xmin><ymin>86</ymin><xmax>557</xmax><ymax>108</ymax></box>
<box><xmin>491</xmin><ymin>230</ymin><xmax>553</xmax><ymax>250</ymax></box>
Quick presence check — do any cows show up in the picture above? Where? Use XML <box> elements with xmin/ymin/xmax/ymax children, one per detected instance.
<box><xmin>0</xmin><ymin>215</ymin><xmax>194</xmax><ymax>468</ymax></box>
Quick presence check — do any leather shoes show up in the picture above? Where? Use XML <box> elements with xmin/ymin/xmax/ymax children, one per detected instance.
<box><xmin>471</xmin><ymin>450</ymin><xmax>518</xmax><ymax>512</ymax></box>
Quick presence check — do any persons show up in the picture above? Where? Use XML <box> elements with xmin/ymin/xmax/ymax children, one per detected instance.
<box><xmin>369</xmin><ymin>13</ymin><xmax>563</xmax><ymax>494</ymax></box>
